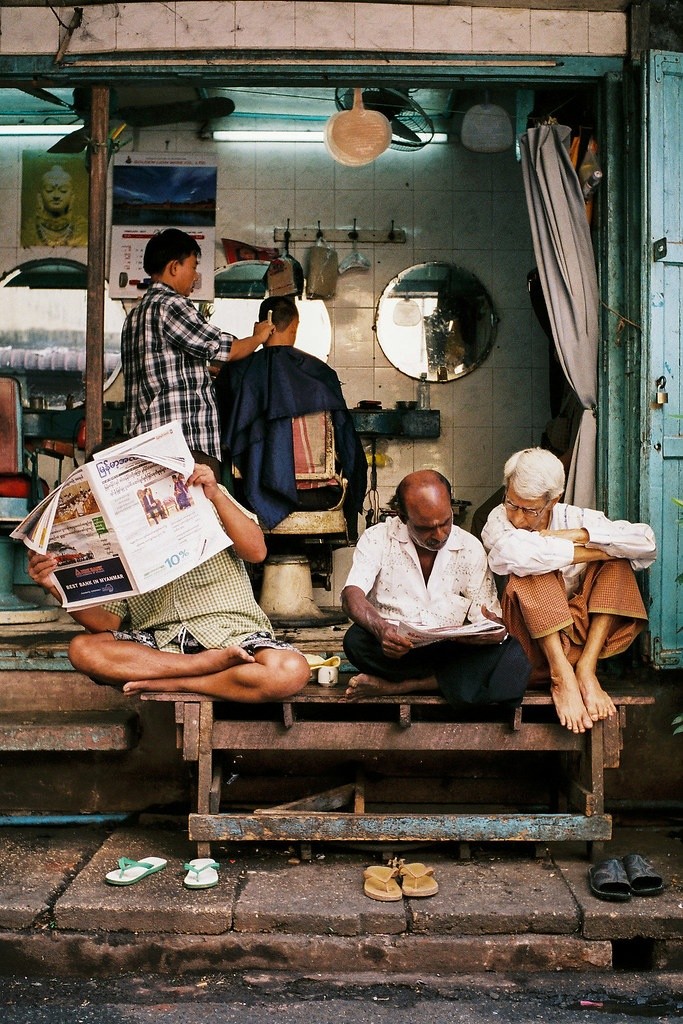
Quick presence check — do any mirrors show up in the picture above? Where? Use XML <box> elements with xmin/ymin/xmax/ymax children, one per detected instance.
<box><xmin>194</xmin><ymin>258</ymin><xmax>334</xmax><ymax>364</ymax></box>
<box><xmin>376</xmin><ymin>261</ymin><xmax>498</xmax><ymax>383</ymax></box>
<box><xmin>0</xmin><ymin>256</ymin><xmax>129</xmax><ymax>410</ymax></box>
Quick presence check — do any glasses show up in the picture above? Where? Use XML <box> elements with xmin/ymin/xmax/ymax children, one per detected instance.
<box><xmin>501</xmin><ymin>483</ymin><xmax>550</xmax><ymax>517</ymax></box>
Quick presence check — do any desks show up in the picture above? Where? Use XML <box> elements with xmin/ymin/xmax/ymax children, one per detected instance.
<box><xmin>19</xmin><ymin>406</ymin><xmax>121</xmax><ymax>443</ymax></box>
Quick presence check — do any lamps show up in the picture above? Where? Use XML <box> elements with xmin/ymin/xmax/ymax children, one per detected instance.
<box><xmin>213</xmin><ymin>130</ymin><xmax>459</xmax><ymax>145</ymax></box>
<box><xmin>0</xmin><ymin>124</ymin><xmax>85</xmax><ymax>135</ymax></box>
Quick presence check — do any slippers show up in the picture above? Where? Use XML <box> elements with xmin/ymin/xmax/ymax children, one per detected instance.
<box><xmin>182</xmin><ymin>857</ymin><xmax>220</xmax><ymax>889</ymax></box>
<box><xmin>588</xmin><ymin>853</ymin><xmax>663</xmax><ymax>901</ymax></box>
<box><xmin>361</xmin><ymin>862</ymin><xmax>439</xmax><ymax>901</ymax></box>
<box><xmin>104</xmin><ymin>857</ymin><xmax>168</xmax><ymax>885</ymax></box>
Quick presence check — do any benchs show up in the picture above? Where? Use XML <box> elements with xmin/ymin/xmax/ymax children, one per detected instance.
<box><xmin>0</xmin><ymin>709</ymin><xmax>141</xmax><ymax>752</ymax></box>
<box><xmin>142</xmin><ymin>689</ymin><xmax>658</xmax><ymax>862</ymax></box>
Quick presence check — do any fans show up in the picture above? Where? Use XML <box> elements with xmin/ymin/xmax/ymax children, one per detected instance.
<box><xmin>335</xmin><ymin>85</ymin><xmax>435</xmax><ymax>152</ymax></box>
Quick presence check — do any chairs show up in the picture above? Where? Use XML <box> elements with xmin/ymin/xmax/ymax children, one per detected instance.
<box><xmin>0</xmin><ymin>375</ymin><xmax>64</xmax><ymax>593</ymax></box>
<box><xmin>228</xmin><ymin>409</ymin><xmax>352</xmax><ymax>629</ymax></box>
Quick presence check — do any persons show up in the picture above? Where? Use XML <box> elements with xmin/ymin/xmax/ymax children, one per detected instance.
<box><xmin>138</xmin><ymin>473</ymin><xmax>189</xmax><ymax>525</ymax></box>
<box><xmin>217</xmin><ymin>296</ymin><xmax>368</xmax><ymax>529</ymax></box>
<box><xmin>120</xmin><ymin>228</ymin><xmax>275</xmax><ymax>460</ymax></box>
<box><xmin>340</xmin><ymin>469</ymin><xmax>532</xmax><ymax>705</ymax></box>
<box><xmin>21</xmin><ymin>166</ymin><xmax>88</xmax><ymax>248</ymax></box>
<box><xmin>482</xmin><ymin>448</ymin><xmax>655</xmax><ymax>734</ymax></box>
<box><xmin>28</xmin><ymin>434</ymin><xmax>309</xmax><ymax>705</ymax></box>
